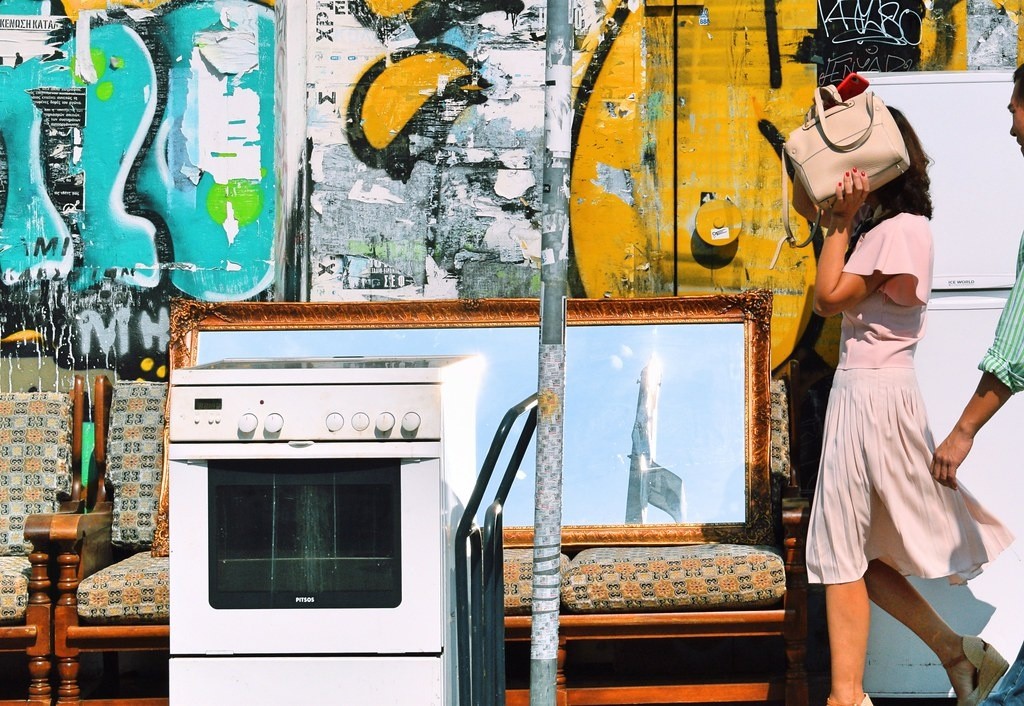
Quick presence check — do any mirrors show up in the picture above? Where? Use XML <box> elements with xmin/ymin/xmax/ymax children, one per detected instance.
<box><xmin>150</xmin><ymin>286</ymin><xmax>775</xmax><ymax>557</ymax></box>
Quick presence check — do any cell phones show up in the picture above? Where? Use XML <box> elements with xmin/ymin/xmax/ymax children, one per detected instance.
<box><xmin>825</xmin><ymin>72</ymin><xmax>869</xmax><ymax>108</ymax></box>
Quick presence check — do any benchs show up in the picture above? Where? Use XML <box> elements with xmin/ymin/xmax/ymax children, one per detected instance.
<box><xmin>50</xmin><ymin>387</ymin><xmax>822</xmax><ymax>706</ymax></box>
<box><xmin>0</xmin><ymin>374</ymin><xmax>89</xmax><ymax>706</ymax></box>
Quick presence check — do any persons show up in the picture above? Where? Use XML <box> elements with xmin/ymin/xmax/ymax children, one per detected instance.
<box><xmin>791</xmin><ymin>106</ymin><xmax>1015</xmax><ymax>706</ymax></box>
<box><xmin>929</xmin><ymin>64</ymin><xmax>1024</xmax><ymax>706</ymax></box>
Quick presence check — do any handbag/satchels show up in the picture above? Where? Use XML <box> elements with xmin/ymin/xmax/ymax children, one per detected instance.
<box><xmin>770</xmin><ymin>84</ymin><xmax>910</xmax><ymax>271</ymax></box>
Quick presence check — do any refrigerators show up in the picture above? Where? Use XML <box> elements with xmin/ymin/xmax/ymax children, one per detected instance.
<box><xmin>856</xmin><ymin>70</ymin><xmax>1024</xmax><ymax>698</ymax></box>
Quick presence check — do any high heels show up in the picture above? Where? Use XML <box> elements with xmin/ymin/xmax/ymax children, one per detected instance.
<box><xmin>943</xmin><ymin>635</ymin><xmax>1009</xmax><ymax>706</ymax></box>
<box><xmin>828</xmin><ymin>693</ymin><xmax>874</xmax><ymax>706</ymax></box>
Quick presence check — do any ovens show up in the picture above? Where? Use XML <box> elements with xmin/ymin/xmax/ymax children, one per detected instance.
<box><xmin>169</xmin><ymin>384</ymin><xmax>483</xmax><ymax>706</ymax></box>
